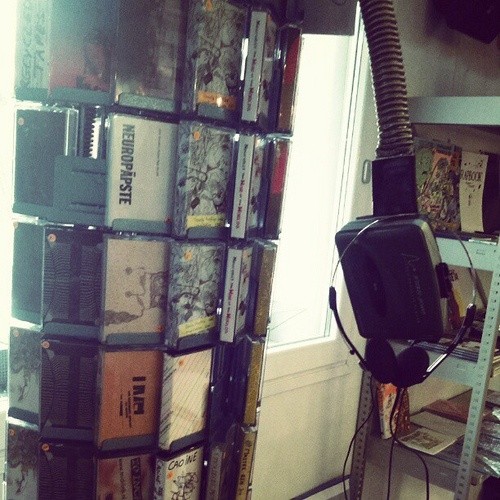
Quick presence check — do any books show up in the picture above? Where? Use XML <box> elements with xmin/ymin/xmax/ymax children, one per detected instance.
<box><xmin>5</xmin><ymin>0</ymin><xmax>303</xmax><ymax>500</ymax></box>
<box><xmin>370</xmin><ymin>138</ymin><xmax>500</xmax><ymax>480</ymax></box>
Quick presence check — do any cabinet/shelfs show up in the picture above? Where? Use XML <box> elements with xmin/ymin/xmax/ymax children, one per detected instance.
<box><xmin>346</xmin><ymin>97</ymin><xmax>500</xmax><ymax>500</ymax></box>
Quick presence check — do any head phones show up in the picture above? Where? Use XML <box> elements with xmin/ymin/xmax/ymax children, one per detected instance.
<box><xmin>328</xmin><ymin>212</ymin><xmax>478</xmax><ymax>389</ymax></box>
